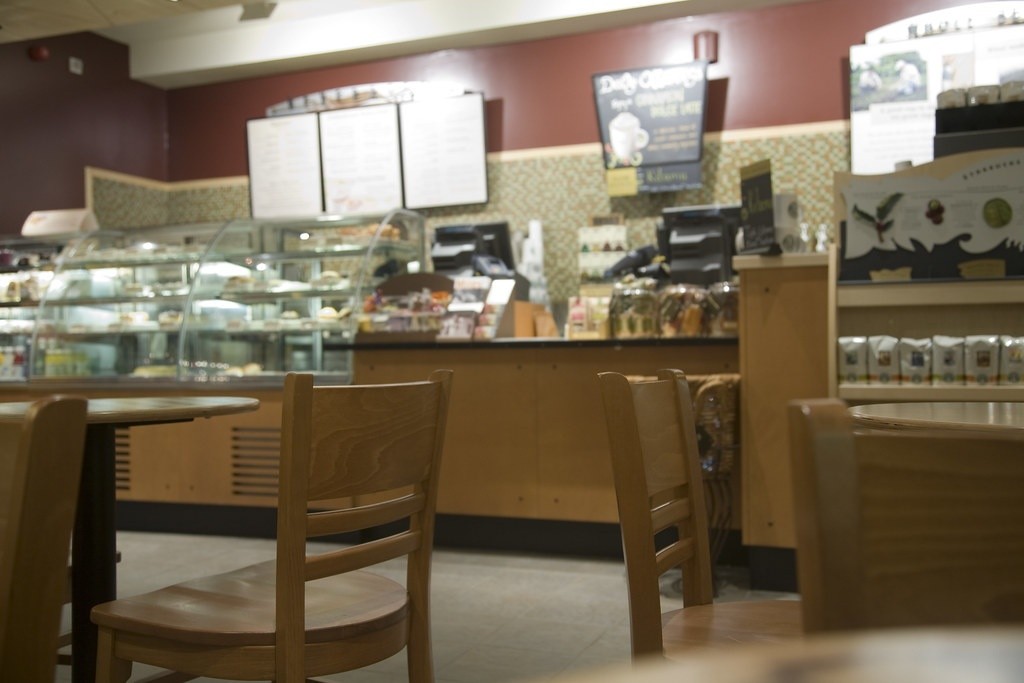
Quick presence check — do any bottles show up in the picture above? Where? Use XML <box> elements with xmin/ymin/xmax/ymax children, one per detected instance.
<box><xmin>0</xmin><ymin>333</ymin><xmax>91</xmax><ymax>381</ymax></box>
<box><xmin>608</xmin><ymin>280</ymin><xmax>740</xmax><ymax>339</ymax></box>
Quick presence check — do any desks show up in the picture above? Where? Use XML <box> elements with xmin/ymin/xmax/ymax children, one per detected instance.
<box><xmin>0</xmin><ymin>397</ymin><xmax>261</xmax><ymax>683</ymax></box>
<box><xmin>849</xmin><ymin>402</ymin><xmax>1024</xmax><ymax>431</ymax></box>
<box><xmin>731</xmin><ymin>254</ymin><xmax>829</xmax><ymax>592</ymax></box>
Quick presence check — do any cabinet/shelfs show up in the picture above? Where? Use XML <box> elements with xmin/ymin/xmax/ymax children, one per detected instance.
<box><xmin>0</xmin><ymin>209</ymin><xmax>425</xmax><ymax>542</ymax></box>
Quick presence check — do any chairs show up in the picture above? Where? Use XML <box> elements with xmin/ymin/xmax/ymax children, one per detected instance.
<box><xmin>593</xmin><ymin>367</ymin><xmax>1024</xmax><ymax>662</ymax></box>
<box><xmin>0</xmin><ymin>368</ymin><xmax>457</xmax><ymax>683</ymax></box>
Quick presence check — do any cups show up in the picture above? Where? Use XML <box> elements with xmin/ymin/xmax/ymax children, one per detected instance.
<box><xmin>608</xmin><ymin>115</ymin><xmax>650</xmax><ymax>161</ymax></box>
